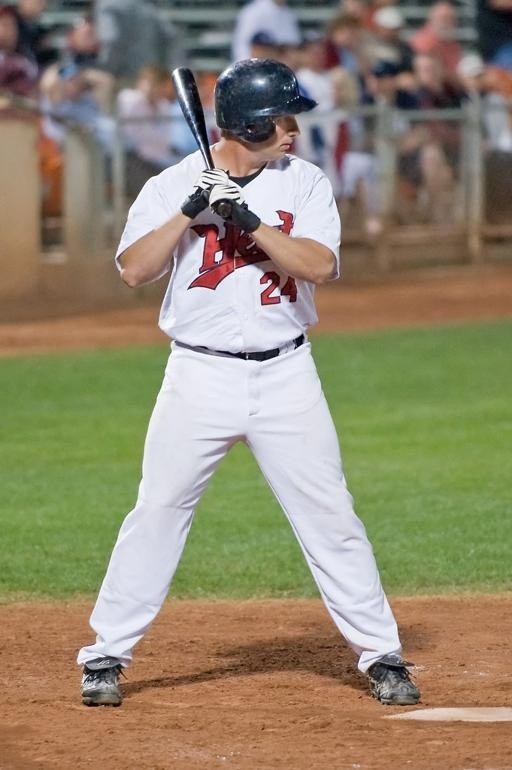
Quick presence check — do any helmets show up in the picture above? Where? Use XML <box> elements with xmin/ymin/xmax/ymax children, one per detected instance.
<box><xmin>216</xmin><ymin>58</ymin><xmax>318</xmax><ymax>142</ymax></box>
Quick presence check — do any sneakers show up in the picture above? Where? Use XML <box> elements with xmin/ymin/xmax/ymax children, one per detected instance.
<box><xmin>81</xmin><ymin>657</ymin><xmax>123</xmax><ymax>707</ymax></box>
<box><xmin>368</xmin><ymin>654</ymin><xmax>419</xmax><ymax>706</ymax></box>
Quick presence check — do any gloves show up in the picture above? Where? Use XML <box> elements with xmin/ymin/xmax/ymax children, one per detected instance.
<box><xmin>174</xmin><ymin>335</ymin><xmax>303</xmax><ymax>361</ymax></box>
<box><xmin>180</xmin><ymin>169</ymin><xmax>230</xmax><ymax>219</ymax></box>
<box><xmin>209</xmin><ymin>185</ymin><xmax>260</xmax><ymax>234</ymax></box>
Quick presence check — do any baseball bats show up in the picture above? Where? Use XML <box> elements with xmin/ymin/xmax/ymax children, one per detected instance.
<box><xmin>172</xmin><ymin>68</ymin><xmax>234</xmax><ymax>220</ymax></box>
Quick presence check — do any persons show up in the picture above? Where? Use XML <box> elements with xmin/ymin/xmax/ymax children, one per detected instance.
<box><xmin>75</xmin><ymin>57</ymin><xmax>421</xmax><ymax>706</ymax></box>
<box><xmin>1</xmin><ymin>1</ymin><xmax>512</xmax><ymax>215</ymax></box>
